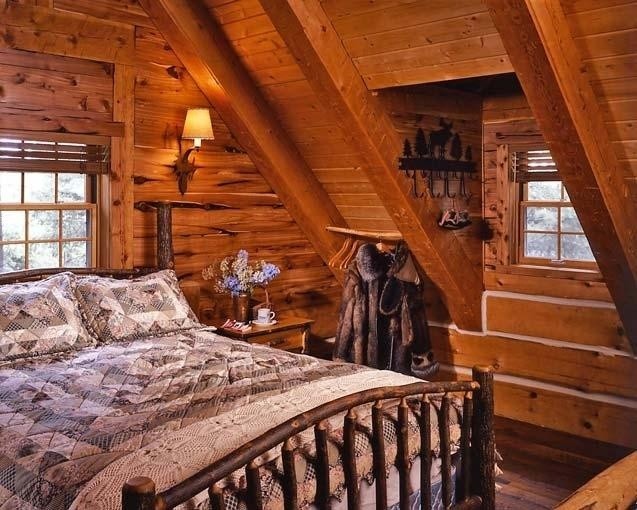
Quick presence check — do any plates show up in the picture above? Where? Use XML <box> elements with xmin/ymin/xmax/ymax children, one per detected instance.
<box><xmin>251</xmin><ymin>319</ymin><xmax>277</xmax><ymax>327</ymax></box>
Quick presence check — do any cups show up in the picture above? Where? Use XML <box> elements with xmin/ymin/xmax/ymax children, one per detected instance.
<box><xmin>257</xmin><ymin>308</ymin><xmax>275</xmax><ymax>324</ymax></box>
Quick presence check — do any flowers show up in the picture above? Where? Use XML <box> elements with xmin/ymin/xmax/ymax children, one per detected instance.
<box><xmin>202</xmin><ymin>250</ymin><xmax>280</xmax><ymax>300</ymax></box>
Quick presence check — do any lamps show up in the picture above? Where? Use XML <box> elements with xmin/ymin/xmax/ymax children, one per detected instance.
<box><xmin>181</xmin><ymin>108</ymin><xmax>215</xmax><ymax>150</ymax></box>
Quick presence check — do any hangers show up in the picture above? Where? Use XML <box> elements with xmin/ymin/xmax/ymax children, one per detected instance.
<box><xmin>328</xmin><ymin>224</ymin><xmax>395</xmax><ymax>272</ymax></box>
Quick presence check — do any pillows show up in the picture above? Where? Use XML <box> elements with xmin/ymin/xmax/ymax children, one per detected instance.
<box><xmin>0</xmin><ymin>270</ymin><xmax>203</xmax><ymax>362</ymax></box>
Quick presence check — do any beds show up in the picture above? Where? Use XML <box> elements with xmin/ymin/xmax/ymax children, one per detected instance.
<box><xmin>0</xmin><ymin>268</ymin><xmax>496</xmax><ymax>510</ymax></box>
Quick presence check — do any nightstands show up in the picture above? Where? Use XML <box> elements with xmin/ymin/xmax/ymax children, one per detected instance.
<box><xmin>200</xmin><ymin>314</ymin><xmax>316</xmax><ymax>356</ymax></box>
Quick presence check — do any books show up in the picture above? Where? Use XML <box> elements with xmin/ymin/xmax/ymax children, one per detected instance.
<box><xmin>220</xmin><ymin>319</ymin><xmax>251</xmax><ymax>332</ymax></box>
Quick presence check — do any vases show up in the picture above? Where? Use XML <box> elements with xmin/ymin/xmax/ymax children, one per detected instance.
<box><xmin>233</xmin><ymin>293</ymin><xmax>252</xmax><ymax>322</ymax></box>
